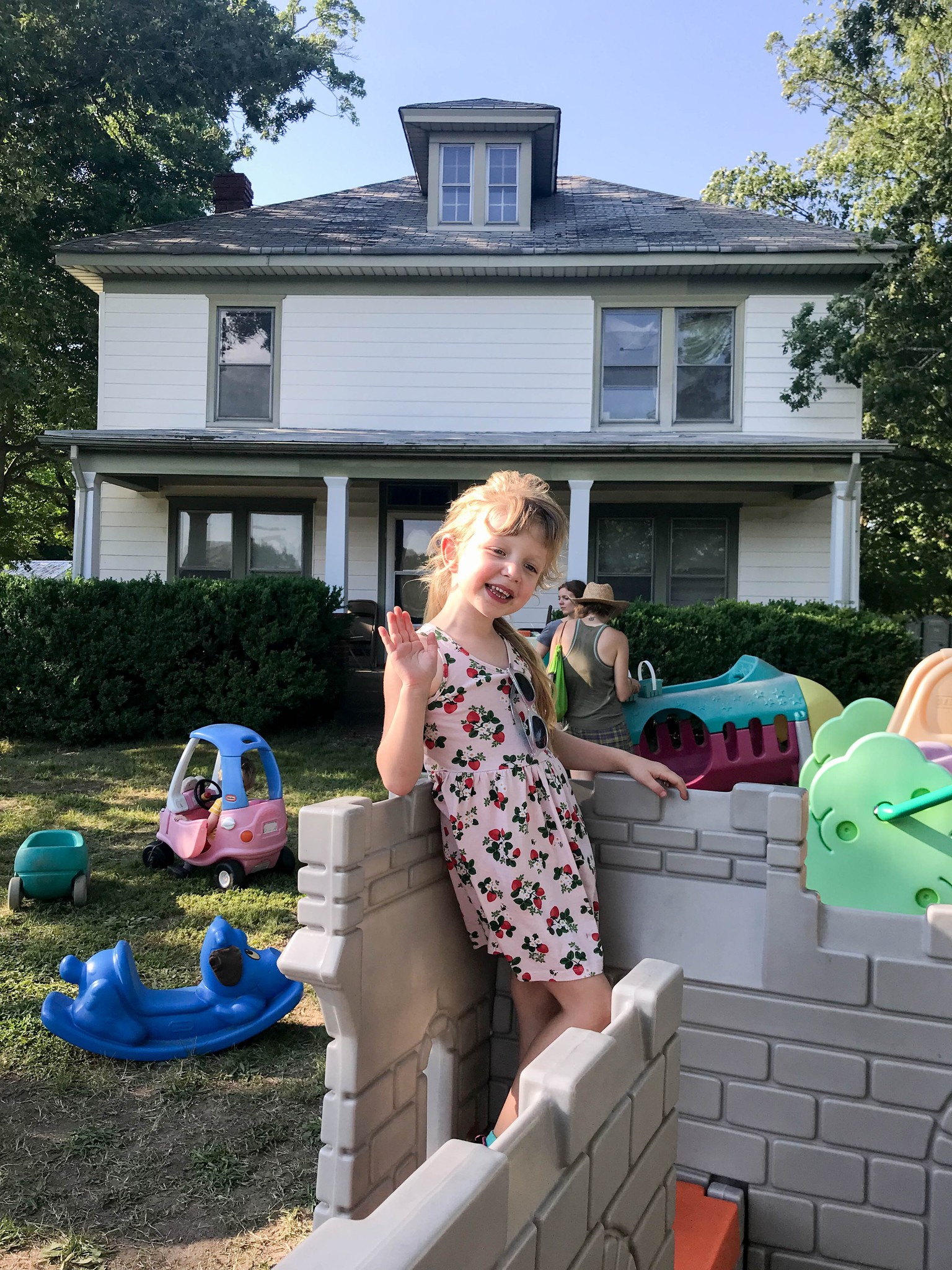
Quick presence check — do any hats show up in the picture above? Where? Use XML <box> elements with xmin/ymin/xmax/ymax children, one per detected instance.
<box><xmin>567</xmin><ymin>582</ymin><xmax>631</xmax><ymax>611</ymax></box>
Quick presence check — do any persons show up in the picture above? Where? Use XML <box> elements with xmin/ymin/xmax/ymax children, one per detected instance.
<box><xmin>173</xmin><ymin>756</ymin><xmax>256</xmax><ymax>853</ymax></box>
<box><xmin>376</xmin><ymin>469</ymin><xmax>689</xmax><ymax>1149</ymax></box>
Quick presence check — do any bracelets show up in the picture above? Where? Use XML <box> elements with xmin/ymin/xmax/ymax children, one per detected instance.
<box><xmin>628</xmin><ymin>677</ymin><xmax>632</xmax><ymax>685</ymax></box>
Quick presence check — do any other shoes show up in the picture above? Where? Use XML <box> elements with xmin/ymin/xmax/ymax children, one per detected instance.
<box><xmin>176</xmin><ymin>864</ymin><xmax>191</xmax><ymax>878</ymax></box>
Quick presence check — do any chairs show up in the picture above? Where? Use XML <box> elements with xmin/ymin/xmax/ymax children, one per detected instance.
<box><xmin>347</xmin><ymin>598</ymin><xmax>378</xmax><ymax>671</ymax></box>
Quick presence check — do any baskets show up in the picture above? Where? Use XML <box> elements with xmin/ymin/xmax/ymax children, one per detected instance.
<box><xmin>637</xmin><ymin>660</ymin><xmax>664</xmax><ymax>698</ymax></box>
<box><xmin>621</xmin><ymin>670</ymin><xmax>638</xmax><ymax>702</ymax></box>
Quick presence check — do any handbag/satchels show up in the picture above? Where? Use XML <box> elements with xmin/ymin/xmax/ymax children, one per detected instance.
<box><xmin>546</xmin><ymin>621</ymin><xmax>568</xmax><ymax>723</ymax></box>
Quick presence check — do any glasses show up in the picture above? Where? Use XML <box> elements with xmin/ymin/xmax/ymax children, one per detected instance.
<box><xmin>505</xmin><ymin>667</ymin><xmax>550</xmax><ymax>758</ymax></box>
